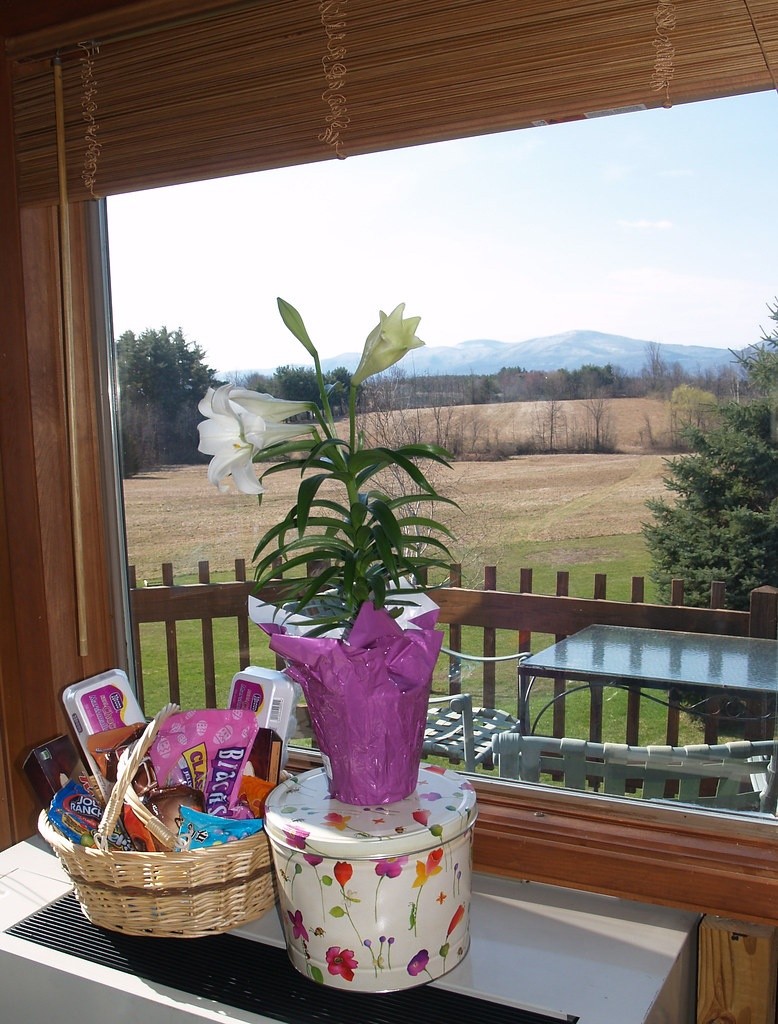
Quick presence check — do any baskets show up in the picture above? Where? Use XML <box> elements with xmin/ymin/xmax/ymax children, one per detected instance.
<box><xmin>38</xmin><ymin>703</ymin><xmax>278</xmax><ymax>938</ymax></box>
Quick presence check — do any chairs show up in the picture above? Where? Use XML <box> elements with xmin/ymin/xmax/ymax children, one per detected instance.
<box><xmin>487</xmin><ymin>731</ymin><xmax>778</xmax><ymax>816</ymax></box>
<box><xmin>415</xmin><ymin>694</ymin><xmax>522</xmax><ymax>779</ymax></box>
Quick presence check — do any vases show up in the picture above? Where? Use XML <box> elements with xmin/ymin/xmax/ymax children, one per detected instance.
<box><xmin>241</xmin><ymin>596</ymin><xmax>445</xmax><ymax>808</ymax></box>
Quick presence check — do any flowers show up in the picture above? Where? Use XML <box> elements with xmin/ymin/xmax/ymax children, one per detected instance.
<box><xmin>194</xmin><ymin>296</ymin><xmax>427</xmax><ymax>647</ymax></box>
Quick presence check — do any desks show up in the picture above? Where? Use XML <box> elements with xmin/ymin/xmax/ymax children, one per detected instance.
<box><xmin>514</xmin><ymin>623</ymin><xmax>778</xmax><ymax>696</ymax></box>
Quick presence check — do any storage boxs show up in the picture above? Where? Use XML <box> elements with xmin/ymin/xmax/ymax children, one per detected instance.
<box><xmin>20</xmin><ymin>734</ymin><xmax>90</xmax><ymax>809</ymax></box>
